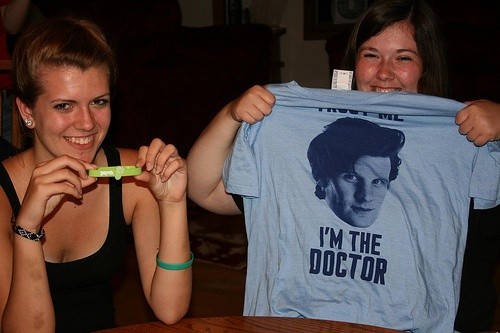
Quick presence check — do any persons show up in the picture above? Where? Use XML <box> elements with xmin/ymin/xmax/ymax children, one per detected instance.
<box><xmin>0</xmin><ymin>16</ymin><xmax>194</xmax><ymax>333</ymax></box>
<box><xmin>307</xmin><ymin>118</ymin><xmax>405</xmax><ymax>228</ymax></box>
<box><xmin>185</xmin><ymin>0</ymin><xmax>500</xmax><ymax>279</ymax></box>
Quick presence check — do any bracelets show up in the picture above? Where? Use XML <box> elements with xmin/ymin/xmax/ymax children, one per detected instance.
<box><xmin>11</xmin><ymin>223</ymin><xmax>45</xmax><ymax>241</ymax></box>
<box><xmin>156</xmin><ymin>250</ymin><xmax>193</xmax><ymax>271</ymax></box>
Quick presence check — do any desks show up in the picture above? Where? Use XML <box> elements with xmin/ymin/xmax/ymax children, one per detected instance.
<box><xmin>89</xmin><ymin>316</ymin><xmax>411</xmax><ymax>333</ymax></box>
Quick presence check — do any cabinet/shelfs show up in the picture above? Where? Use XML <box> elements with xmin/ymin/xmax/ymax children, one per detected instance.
<box><xmin>104</xmin><ymin>24</ymin><xmax>287</xmax><ymax>222</ymax></box>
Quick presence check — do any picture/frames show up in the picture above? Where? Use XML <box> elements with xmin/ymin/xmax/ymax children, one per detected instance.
<box><xmin>304</xmin><ymin>0</ymin><xmax>377</xmax><ymax>41</ymax></box>
